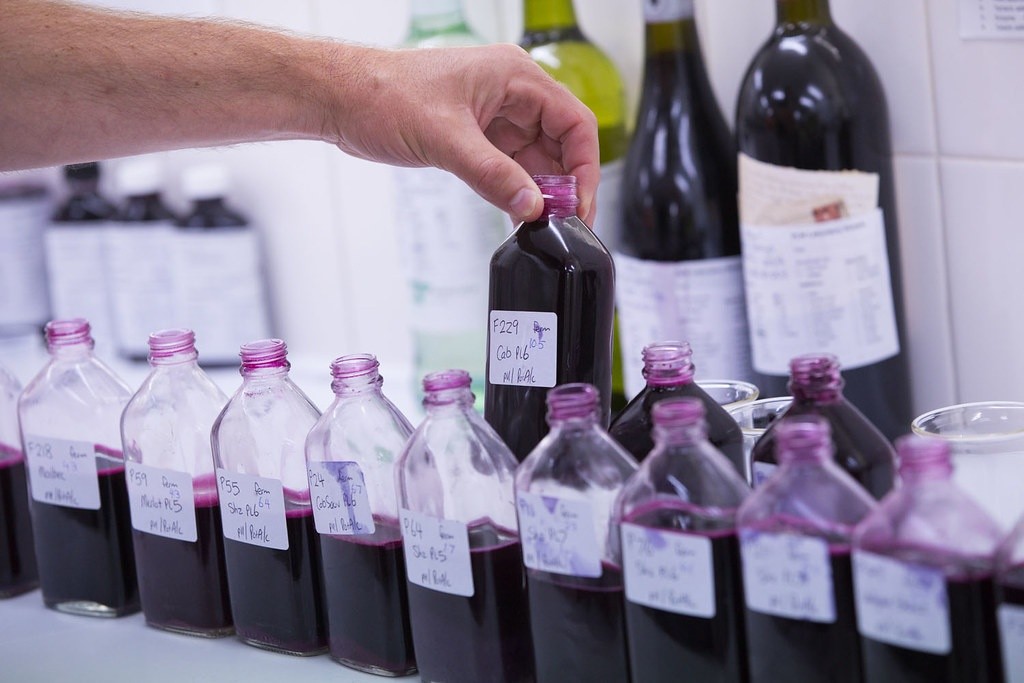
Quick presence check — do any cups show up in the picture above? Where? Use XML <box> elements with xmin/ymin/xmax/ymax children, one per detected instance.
<box><xmin>724</xmin><ymin>395</ymin><xmax>795</xmax><ymax>487</ymax></box>
<box><xmin>910</xmin><ymin>401</ymin><xmax>1023</xmax><ymax>533</ymax></box>
<box><xmin>683</xmin><ymin>381</ymin><xmax>760</xmax><ymax>488</ymax></box>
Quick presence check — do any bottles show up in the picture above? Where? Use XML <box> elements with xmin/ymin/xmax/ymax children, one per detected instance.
<box><xmin>4</xmin><ymin>1</ymin><xmax>1024</xmax><ymax>680</ymax></box>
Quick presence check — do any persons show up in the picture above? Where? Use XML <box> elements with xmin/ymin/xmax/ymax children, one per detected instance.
<box><xmin>0</xmin><ymin>1</ymin><xmax>600</xmax><ymax>241</ymax></box>
